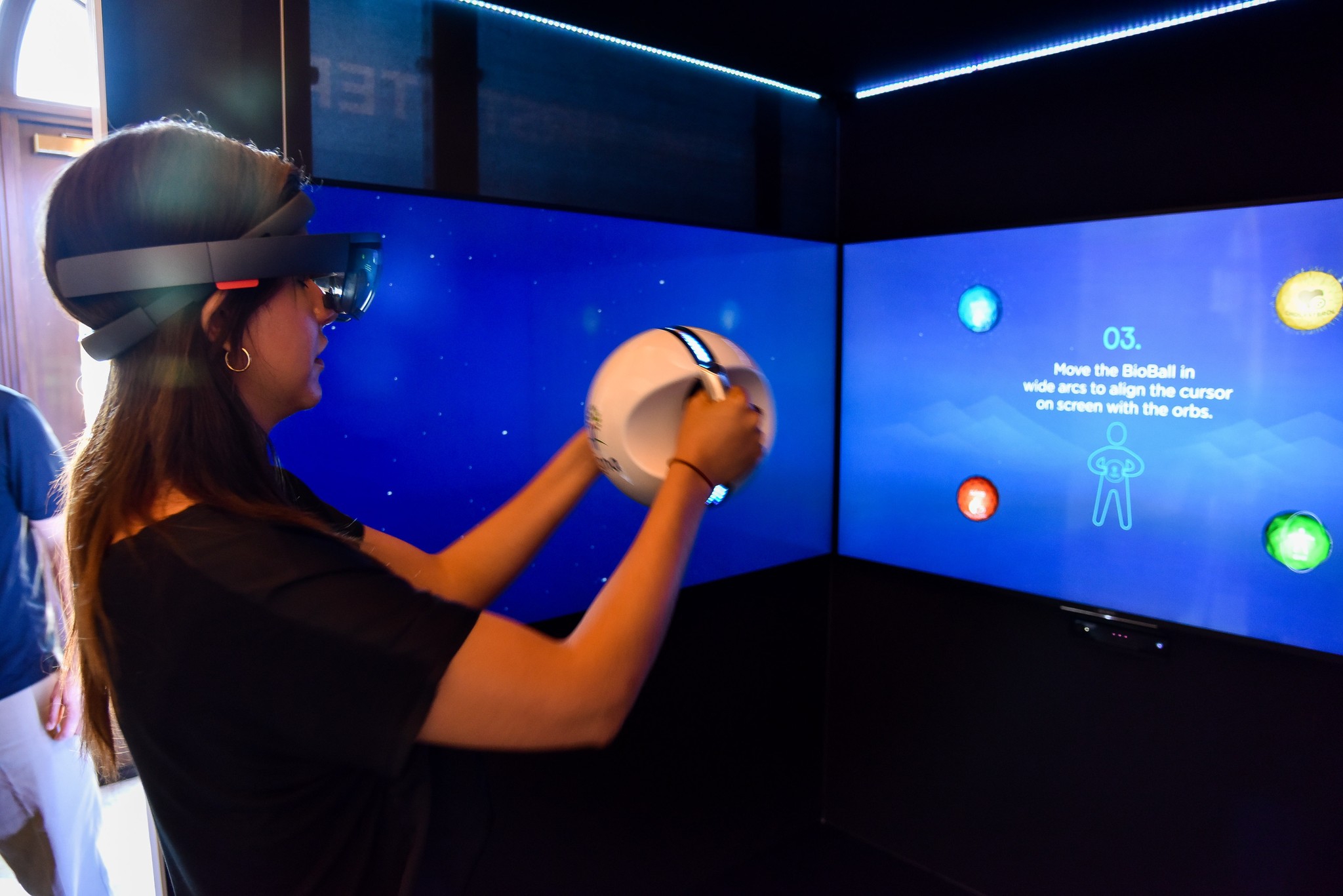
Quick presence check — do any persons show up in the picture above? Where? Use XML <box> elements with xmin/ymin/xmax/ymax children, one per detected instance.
<box><xmin>0</xmin><ymin>385</ymin><xmax>114</xmax><ymax>896</ymax></box>
<box><xmin>44</xmin><ymin>117</ymin><xmax>766</xmax><ymax>895</ymax></box>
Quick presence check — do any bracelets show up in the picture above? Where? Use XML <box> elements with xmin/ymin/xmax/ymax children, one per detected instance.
<box><xmin>668</xmin><ymin>457</ymin><xmax>715</xmax><ymax>491</ymax></box>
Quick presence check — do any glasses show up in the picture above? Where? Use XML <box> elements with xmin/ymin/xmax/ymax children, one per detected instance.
<box><xmin>314</xmin><ymin>232</ymin><xmax>382</xmax><ymax>319</ymax></box>
